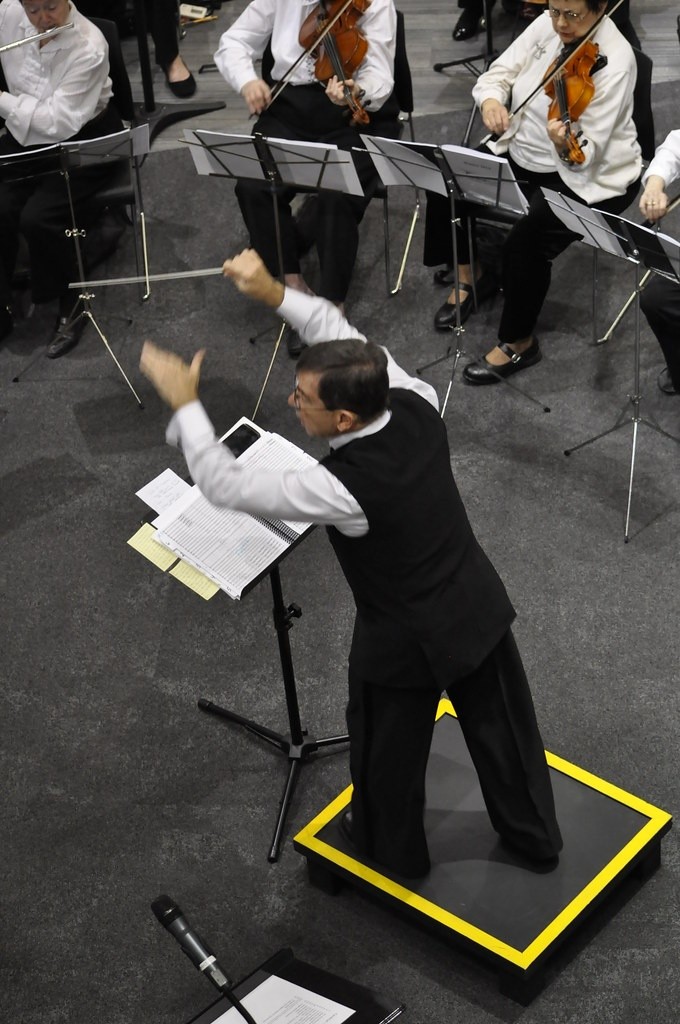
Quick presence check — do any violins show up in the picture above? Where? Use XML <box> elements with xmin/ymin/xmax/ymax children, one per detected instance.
<box><xmin>540</xmin><ymin>39</ymin><xmax>600</xmax><ymax>167</ymax></box>
<box><xmin>298</xmin><ymin>0</ymin><xmax>374</xmax><ymax>127</ymax></box>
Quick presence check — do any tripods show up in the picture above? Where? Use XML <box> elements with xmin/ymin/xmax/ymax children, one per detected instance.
<box><xmin>139</xmin><ymin>422</ymin><xmax>352</xmax><ymax>862</ymax></box>
<box><xmin>0</xmin><ymin>137</ymin><xmax>145</xmax><ymax>409</ymax></box>
<box><xmin>177</xmin><ymin>131</ymin><xmax>349</xmax><ymax>423</ymax></box>
<box><xmin>350</xmin><ymin>136</ymin><xmax>551</xmax><ymax>420</ymax></box>
<box><xmin>544</xmin><ymin>191</ymin><xmax>680</xmax><ymax>544</ymax></box>
<box><xmin>434</xmin><ymin>0</ymin><xmax>505</xmax><ymax>147</ymax></box>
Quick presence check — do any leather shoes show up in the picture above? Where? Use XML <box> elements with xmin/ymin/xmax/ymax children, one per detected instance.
<box><xmin>434</xmin><ymin>268</ymin><xmax>495</xmax><ymax>329</ymax></box>
<box><xmin>453</xmin><ymin>0</ymin><xmax>497</xmax><ymax>41</ymax></box>
<box><xmin>0</xmin><ymin>305</ymin><xmax>14</xmax><ymax>343</ymax></box>
<box><xmin>46</xmin><ymin>302</ymin><xmax>92</xmax><ymax>359</ymax></box>
<box><xmin>463</xmin><ymin>336</ymin><xmax>543</xmax><ymax>384</ymax></box>
<box><xmin>340</xmin><ymin>810</ymin><xmax>357</xmax><ymax>846</ymax></box>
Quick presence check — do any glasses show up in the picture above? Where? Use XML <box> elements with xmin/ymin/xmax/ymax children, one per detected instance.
<box><xmin>293</xmin><ymin>376</ymin><xmax>329</xmax><ymax>411</ymax></box>
<box><xmin>549</xmin><ymin>9</ymin><xmax>590</xmax><ymax>22</ymax></box>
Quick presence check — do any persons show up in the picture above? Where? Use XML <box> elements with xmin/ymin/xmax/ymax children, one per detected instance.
<box><xmin>0</xmin><ymin>0</ymin><xmax>680</xmax><ymax>385</ymax></box>
<box><xmin>139</xmin><ymin>251</ymin><xmax>563</xmax><ymax>878</ymax></box>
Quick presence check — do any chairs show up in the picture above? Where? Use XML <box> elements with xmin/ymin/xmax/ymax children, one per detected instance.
<box><xmin>587</xmin><ymin>44</ymin><xmax>657</xmax><ymax>346</ymax></box>
<box><xmin>78</xmin><ymin>18</ymin><xmax>146</xmax><ymax>304</ymax></box>
<box><xmin>370</xmin><ymin>9</ymin><xmax>421</xmax><ymax>298</ymax></box>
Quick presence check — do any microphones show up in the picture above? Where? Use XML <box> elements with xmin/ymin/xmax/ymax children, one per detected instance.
<box><xmin>151</xmin><ymin>893</ymin><xmax>232</xmax><ymax>990</ymax></box>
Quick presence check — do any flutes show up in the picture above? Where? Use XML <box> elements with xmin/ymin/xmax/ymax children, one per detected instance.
<box><xmin>0</xmin><ymin>22</ymin><xmax>75</xmax><ymax>52</ymax></box>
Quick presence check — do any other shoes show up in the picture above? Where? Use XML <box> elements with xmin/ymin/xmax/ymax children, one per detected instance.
<box><xmin>287</xmin><ymin>330</ymin><xmax>306</xmax><ymax>356</ymax></box>
<box><xmin>160</xmin><ymin>54</ymin><xmax>197</xmax><ymax>98</ymax></box>
